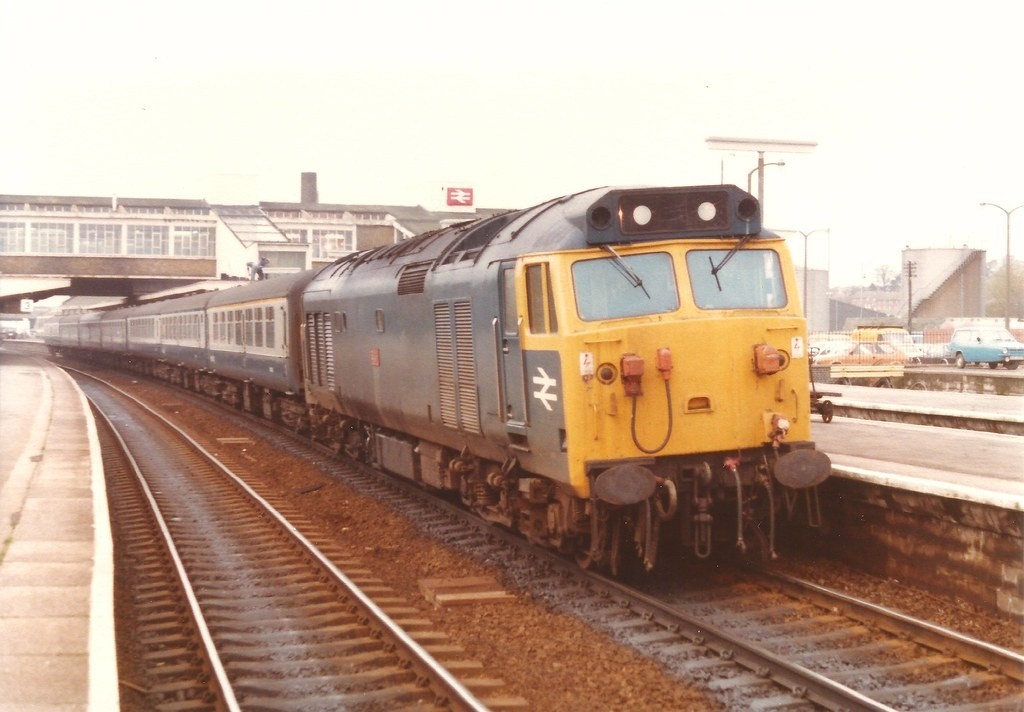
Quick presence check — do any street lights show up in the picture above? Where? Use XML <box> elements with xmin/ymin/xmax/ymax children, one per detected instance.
<box><xmin>980</xmin><ymin>202</ymin><xmax>1024</xmax><ymax>332</ymax></box>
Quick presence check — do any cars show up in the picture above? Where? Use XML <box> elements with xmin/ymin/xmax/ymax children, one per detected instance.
<box><xmin>808</xmin><ymin>326</ymin><xmax>916</xmax><ymax>367</ymax></box>
<box><xmin>949</xmin><ymin>327</ymin><xmax>1024</xmax><ymax>369</ymax></box>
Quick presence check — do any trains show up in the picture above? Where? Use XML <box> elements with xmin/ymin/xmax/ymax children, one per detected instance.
<box><xmin>45</xmin><ymin>184</ymin><xmax>831</xmax><ymax>576</ymax></box>
